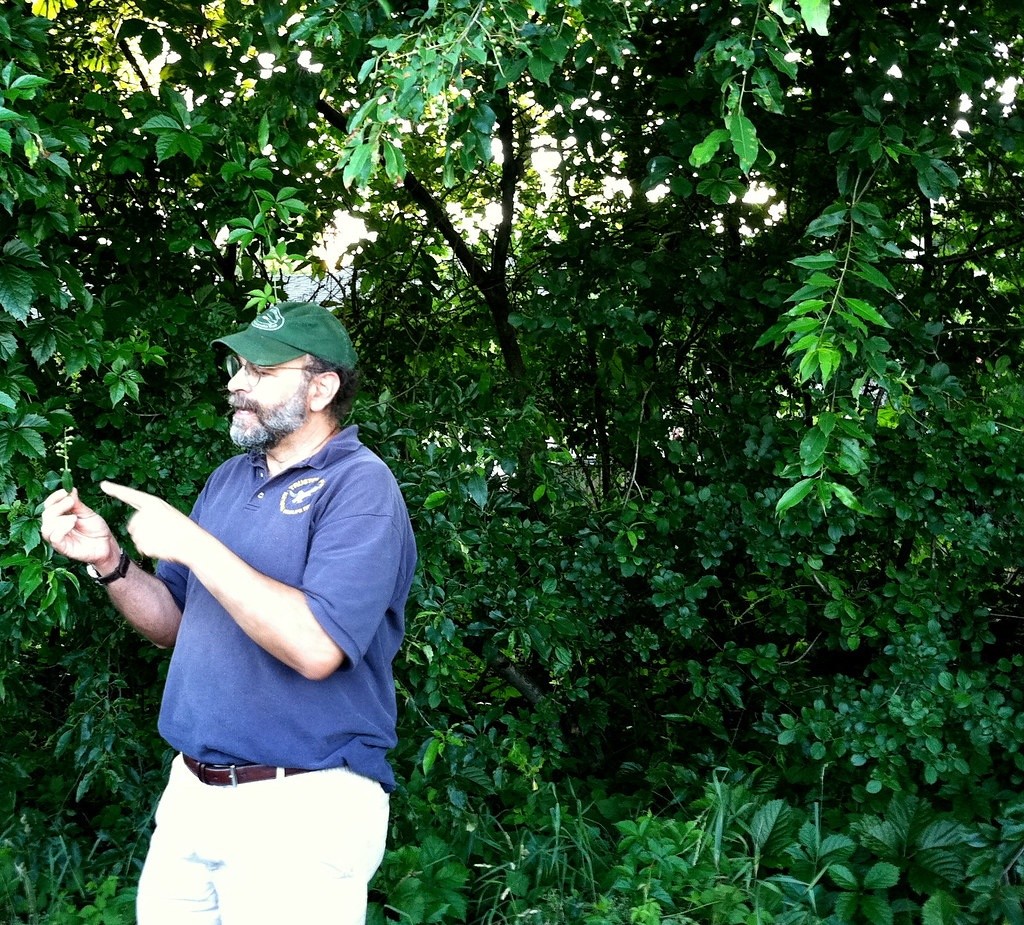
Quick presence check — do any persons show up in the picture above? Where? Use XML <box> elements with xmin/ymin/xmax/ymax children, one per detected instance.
<box><xmin>39</xmin><ymin>301</ymin><xmax>417</xmax><ymax>925</ymax></box>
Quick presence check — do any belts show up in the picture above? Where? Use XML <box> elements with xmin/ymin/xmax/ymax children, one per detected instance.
<box><xmin>182</xmin><ymin>751</ymin><xmax>312</xmax><ymax>787</ymax></box>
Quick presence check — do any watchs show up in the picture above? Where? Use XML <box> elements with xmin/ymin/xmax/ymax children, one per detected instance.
<box><xmin>87</xmin><ymin>548</ymin><xmax>130</xmax><ymax>587</ymax></box>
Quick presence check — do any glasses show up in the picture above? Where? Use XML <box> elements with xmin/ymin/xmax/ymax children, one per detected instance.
<box><xmin>225</xmin><ymin>354</ymin><xmax>325</xmax><ymax>388</ymax></box>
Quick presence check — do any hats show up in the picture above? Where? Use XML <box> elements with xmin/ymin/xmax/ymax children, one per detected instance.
<box><xmin>210</xmin><ymin>302</ymin><xmax>358</xmax><ymax>371</ymax></box>
